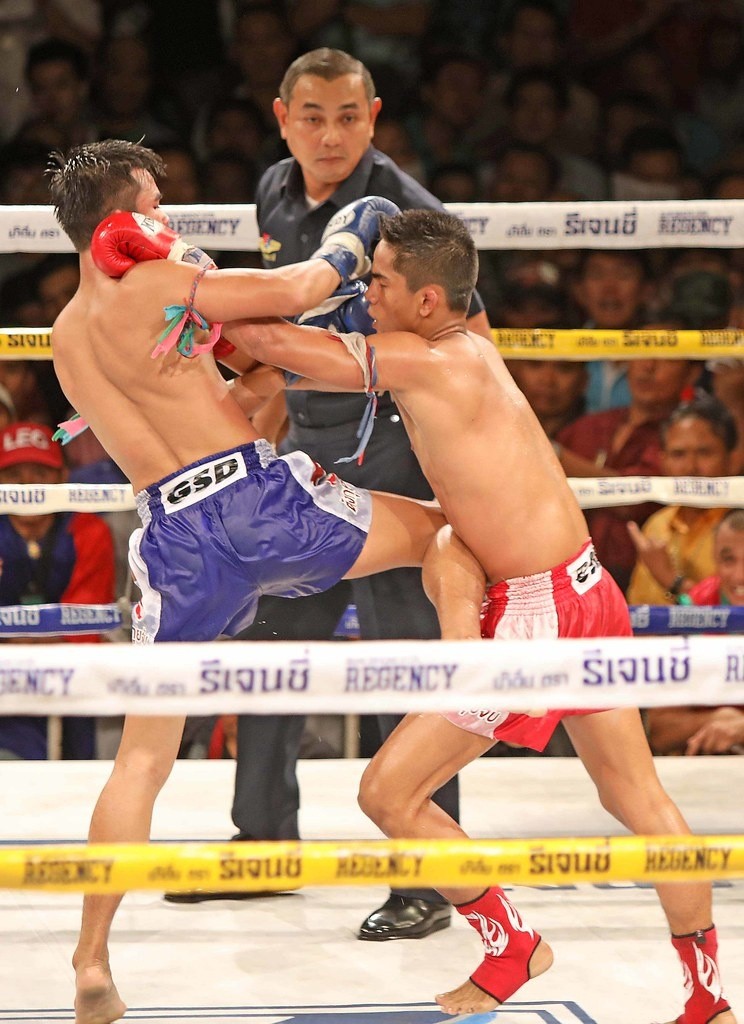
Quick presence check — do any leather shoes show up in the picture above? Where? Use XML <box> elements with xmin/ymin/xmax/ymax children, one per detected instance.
<box><xmin>162</xmin><ymin>833</ymin><xmax>301</xmax><ymax>903</ymax></box>
<box><xmin>359</xmin><ymin>892</ymin><xmax>452</xmax><ymax>941</ymax></box>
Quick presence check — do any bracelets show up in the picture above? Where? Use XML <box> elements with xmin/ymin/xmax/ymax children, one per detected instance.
<box><xmin>552</xmin><ymin>441</ymin><xmax>564</xmax><ymax>460</ymax></box>
<box><xmin>668</xmin><ymin>570</ymin><xmax>690</xmax><ymax>596</ymax></box>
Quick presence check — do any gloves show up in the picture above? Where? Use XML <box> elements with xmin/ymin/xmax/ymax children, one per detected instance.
<box><xmin>309</xmin><ymin>196</ymin><xmax>404</xmax><ymax>288</ymax></box>
<box><xmin>212</xmin><ymin>337</ymin><xmax>238</xmax><ymax>362</ymax></box>
<box><xmin>281</xmin><ymin>279</ymin><xmax>369</xmax><ymax>387</ymax></box>
<box><xmin>92</xmin><ymin>211</ymin><xmax>219</xmax><ymax>280</ymax></box>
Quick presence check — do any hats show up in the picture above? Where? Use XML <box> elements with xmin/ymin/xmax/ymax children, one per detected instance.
<box><xmin>0</xmin><ymin>422</ymin><xmax>64</xmax><ymax>471</ymax></box>
<box><xmin>0</xmin><ymin>382</ymin><xmax>14</xmax><ymax>419</ymax></box>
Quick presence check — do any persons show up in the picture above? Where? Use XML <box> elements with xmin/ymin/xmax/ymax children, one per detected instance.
<box><xmin>89</xmin><ymin>207</ymin><xmax>744</xmax><ymax>1024</ymax></box>
<box><xmin>0</xmin><ymin>0</ymin><xmax>744</xmax><ymax>761</ymax></box>
<box><xmin>49</xmin><ymin>139</ymin><xmax>485</xmax><ymax>1024</ymax></box>
<box><xmin>165</xmin><ymin>46</ymin><xmax>496</xmax><ymax>943</ymax></box>
